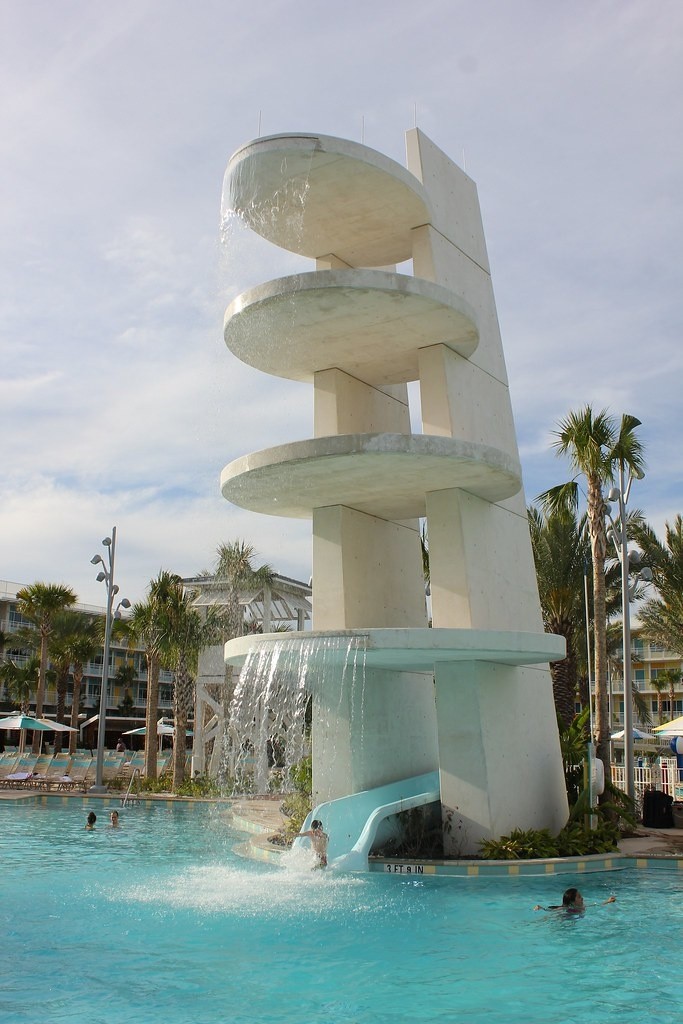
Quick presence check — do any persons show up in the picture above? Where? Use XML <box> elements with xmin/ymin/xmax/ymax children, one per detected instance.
<box><xmin>291</xmin><ymin>820</ymin><xmax>330</xmax><ymax>871</ymax></box>
<box><xmin>0</xmin><ymin>714</ymin><xmax>52</xmax><ymax>752</ymax></box>
<box><xmin>110</xmin><ymin>810</ymin><xmax>119</xmax><ymax>824</ymax></box>
<box><xmin>533</xmin><ymin>888</ymin><xmax>615</xmax><ymax>910</ymax></box>
<box><xmin>638</xmin><ymin>754</ymin><xmax>643</xmax><ymax>767</ymax></box>
<box><xmin>85</xmin><ymin>812</ymin><xmax>96</xmax><ymax>829</ymax></box>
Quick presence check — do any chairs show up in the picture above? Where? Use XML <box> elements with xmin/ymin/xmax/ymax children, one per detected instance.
<box><xmin>0</xmin><ymin>749</ymin><xmax>192</xmax><ymax>792</ymax></box>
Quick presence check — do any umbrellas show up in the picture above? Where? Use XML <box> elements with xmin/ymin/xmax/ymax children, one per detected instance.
<box><xmin>611</xmin><ymin>727</ymin><xmax>656</xmax><ymax>739</ymax></box>
<box><xmin>122</xmin><ymin>725</ymin><xmax>174</xmax><ymax>753</ymax></box>
<box><xmin>31</xmin><ymin>718</ymin><xmax>79</xmax><ymax>754</ymax></box>
<box><xmin>654</xmin><ymin>731</ymin><xmax>683</xmax><ymax>736</ymax></box>
<box><xmin>652</xmin><ymin>715</ymin><xmax>683</xmax><ymax>731</ymax></box>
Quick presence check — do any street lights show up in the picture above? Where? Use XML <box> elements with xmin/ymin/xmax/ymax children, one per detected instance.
<box><xmin>606</xmin><ymin>464</ymin><xmax>654</xmax><ymax>826</ymax></box>
<box><xmin>86</xmin><ymin>526</ymin><xmax>131</xmax><ymax>792</ymax></box>
